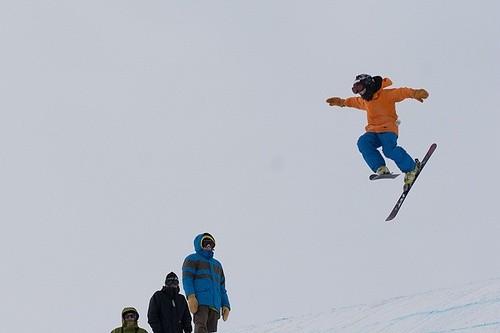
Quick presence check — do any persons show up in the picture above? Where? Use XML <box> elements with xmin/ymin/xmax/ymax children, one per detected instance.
<box><xmin>111</xmin><ymin>307</ymin><xmax>148</xmax><ymax>333</ymax></box>
<box><xmin>182</xmin><ymin>233</ymin><xmax>231</xmax><ymax>333</ymax></box>
<box><xmin>325</xmin><ymin>74</ymin><xmax>429</xmax><ymax>183</ymax></box>
<box><xmin>147</xmin><ymin>272</ymin><xmax>192</xmax><ymax>333</ymax></box>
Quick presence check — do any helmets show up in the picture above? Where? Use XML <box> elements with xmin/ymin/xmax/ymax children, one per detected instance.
<box><xmin>352</xmin><ymin>74</ymin><xmax>375</xmax><ymax>100</ymax></box>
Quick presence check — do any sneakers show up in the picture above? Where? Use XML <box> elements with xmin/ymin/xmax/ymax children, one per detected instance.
<box><xmin>404</xmin><ymin>165</ymin><xmax>418</xmax><ymax>185</ymax></box>
<box><xmin>373</xmin><ymin>167</ymin><xmax>390</xmax><ymax>175</ymax></box>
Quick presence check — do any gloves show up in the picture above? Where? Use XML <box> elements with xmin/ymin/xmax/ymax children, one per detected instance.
<box><xmin>188</xmin><ymin>294</ymin><xmax>198</xmax><ymax>313</ymax></box>
<box><xmin>325</xmin><ymin>97</ymin><xmax>343</xmax><ymax>107</ymax></box>
<box><xmin>222</xmin><ymin>306</ymin><xmax>229</xmax><ymax>321</ymax></box>
<box><xmin>414</xmin><ymin>90</ymin><xmax>429</xmax><ymax>103</ymax></box>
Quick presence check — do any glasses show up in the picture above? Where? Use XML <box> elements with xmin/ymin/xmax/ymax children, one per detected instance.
<box><xmin>202</xmin><ymin>240</ymin><xmax>214</xmax><ymax>247</ymax></box>
<box><xmin>124</xmin><ymin>314</ymin><xmax>136</xmax><ymax>318</ymax></box>
<box><xmin>166</xmin><ymin>280</ymin><xmax>179</xmax><ymax>286</ymax></box>
<box><xmin>351</xmin><ymin>83</ymin><xmax>364</xmax><ymax>94</ymax></box>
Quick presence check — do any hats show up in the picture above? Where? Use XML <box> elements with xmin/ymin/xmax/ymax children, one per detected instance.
<box><xmin>200</xmin><ymin>235</ymin><xmax>215</xmax><ymax>249</ymax></box>
<box><xmin>165</xmin><ymin>272</ymin><xmax>178</xmax><ymax>283</ymax></box>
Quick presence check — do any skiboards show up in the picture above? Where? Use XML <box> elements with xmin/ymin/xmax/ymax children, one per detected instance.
<box><xmin>369</xmin><ymin>142</ymin><xmax>437</xmax><ymax>221</ymax></box>
